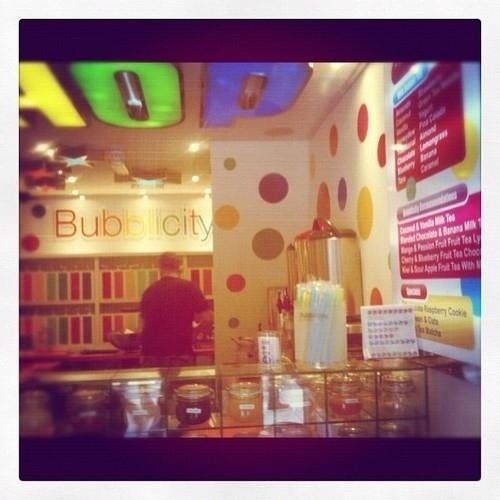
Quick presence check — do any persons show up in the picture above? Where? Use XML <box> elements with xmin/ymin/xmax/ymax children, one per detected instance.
<box><xmin>139</xmin><ymin>253</ymin><xmax>211</xmax><ymax>367</ymax></box>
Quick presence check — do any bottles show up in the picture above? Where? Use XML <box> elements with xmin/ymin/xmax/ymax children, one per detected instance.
<box><xmin>285</xmin><ymin>219</ymin><xmax>364</xmax><ymax>324</ymax></box>
<box><xmin>21</xmin><ymin>362</ymin><xmax>421</xmax><ymax>438</ymax></box>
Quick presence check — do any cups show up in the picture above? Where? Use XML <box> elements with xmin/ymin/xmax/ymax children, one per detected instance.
<box><xmin>294</xmin><ymin>282</ymin><xmax>348</xmax><ymax>368</ymax></box>
<box><xmin>256</xmin><ymin>331</ymin><xmax>282</xmax><ymax>368</ymax></box>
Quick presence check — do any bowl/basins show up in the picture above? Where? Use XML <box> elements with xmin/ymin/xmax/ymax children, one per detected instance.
<box><xmin>108</xmin><ymin>334</ymin><xmax>143</xmax><ymax>352</ymax></box>
